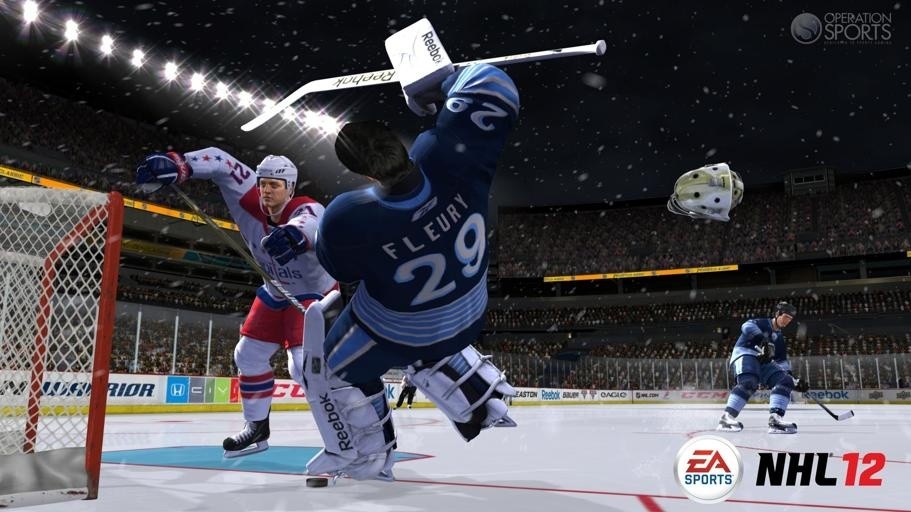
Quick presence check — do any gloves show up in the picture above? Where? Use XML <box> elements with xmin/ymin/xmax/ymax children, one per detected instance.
<box><xmin>756</xmin><ymin>341</ymin><xmax>776</xmax><ymax>365</ymax></box>
<box><xmin>260</xmin><ymin>225</ymin><xmax>310</xmax><ymax>267</ymax></box>
<box><xmin>794</xmin><ymin>377</ymin><xmax>809</xmax><ymax>392</ymax></box>
<box><xmin>135</xmin><ymin>150</ymin><xmax>193</xmax><ymax>198</ymax></box>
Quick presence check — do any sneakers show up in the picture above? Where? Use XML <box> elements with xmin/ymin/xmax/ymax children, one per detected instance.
<box><xmin>768</xmin><ymin>413</ymin><xmax>797</xmax><ymax>431</ymax></box>
<box><xmin>720</xmin><ymin>413</ymin><xmax>744</xmax><ymax>430</ymax></box>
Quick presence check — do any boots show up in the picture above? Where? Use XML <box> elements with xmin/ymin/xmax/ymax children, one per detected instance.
<box><xmin>222</xmin><ymin>404</ymin><xmax>271</xmax><ymax>452</ymax></box>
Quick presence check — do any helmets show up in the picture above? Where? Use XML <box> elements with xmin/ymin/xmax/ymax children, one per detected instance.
<box><xmin>667</xmin><ymin>162</ymin><xmax>745</xmax><ymax>223</ymax></box>
<box><xmin>776</xmin><ymin>301</ymin><xmax>798</xmax><ymax>319</ymax></box>
<box><xmin>256</xmin><ymin>154</ymin><xmax>299</xmax><ymax>194</ymax></box>
